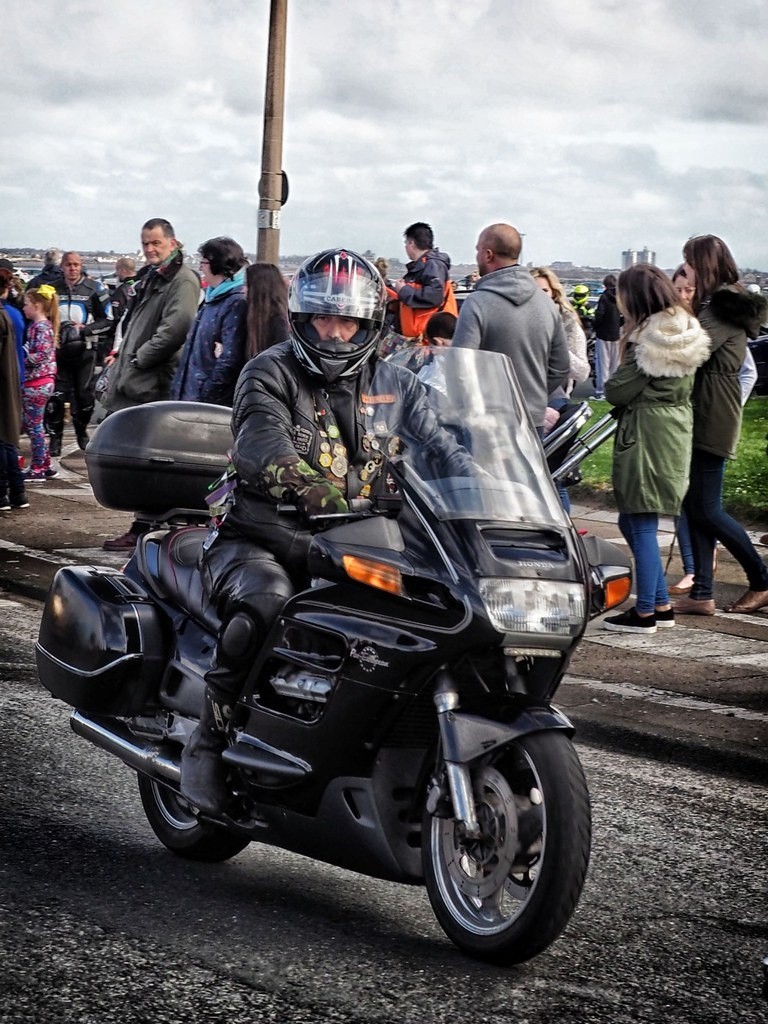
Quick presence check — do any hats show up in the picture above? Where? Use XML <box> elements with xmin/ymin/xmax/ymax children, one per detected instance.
<box><xmin>0</xmin><ymin>258</ymin><xmax>18</xmax><ymax>274</ymax></box>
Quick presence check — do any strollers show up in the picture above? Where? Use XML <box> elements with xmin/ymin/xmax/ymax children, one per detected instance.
<box><xmin>541</xmin><ymin>400</ymin><xmax>620</xmax><ymax>484</ymax></box>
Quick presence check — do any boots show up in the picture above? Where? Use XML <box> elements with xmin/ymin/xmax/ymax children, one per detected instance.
<box><xmin>181</xmin><ymin>688</ymin><xmax>233</xmax><ymax>816</ymax></box>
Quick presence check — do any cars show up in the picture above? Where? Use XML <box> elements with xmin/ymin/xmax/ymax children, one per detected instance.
<box><xmin>561</xmin><ymin>282</ymin><xmax>606</xmax><ymax>316</ymax></box>
<box><xmin>13</xmin><ymin>268</ymin><xmax>42</xmax><ymax>284</ymax></box>
<box><xmin>747</xmin><ymin>324</ymin><xmax>768</xmax><ymax>395</ymax></box>
<box><xmin>453</xmin><ymin>279</ymin><xmax>476</xmax><ymax>299</ymax></box>
<box><xmin>95</xmin><ymin>271</ymin><xmax>120</xmax><ymax>288</ymax></box>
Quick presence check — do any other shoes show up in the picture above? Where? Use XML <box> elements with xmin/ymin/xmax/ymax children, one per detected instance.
<box><xmin>675</xmin><ymin>597</ymin><xmax>717</xmax><ymax>616</ymax></box>
<box><xmin>77</xmin><ymin>432</ymin><xmax>90</xmax><ymax>450</ymax></box>
<box><xmin>24</xmin><ymin>468</ymin><xmax>60</xmax><ymax>482</ymax></box>
<box><xmin>723</xmin><ymin>591</ymin><xmax>768</xmax><ymax>612</ymax></box>
<box><xmin>0</xmin><ymin>494</ymin><xmax>30</xmax><ymax>510</ymax></box>
<box><xmin>668</xmin><ymin>574</ymin><xmax>696</xmax><ymax>595</ymax></box>
<box><xmin>49</xmin><ymin>434</ymin><xmax>63</xmax><ymax>456</ymax></box>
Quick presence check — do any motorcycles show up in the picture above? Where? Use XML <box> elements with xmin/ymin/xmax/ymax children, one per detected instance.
<box><xmin>32</xmin><ymin>347</ymin><xmax>632</xmax><ymax>965</ymax></box>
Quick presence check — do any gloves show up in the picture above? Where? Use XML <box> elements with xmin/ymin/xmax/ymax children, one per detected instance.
<box><xmin>259</xmin><ymin>458</ymin><xmax>349</xmax><ymax>528</ymax></box>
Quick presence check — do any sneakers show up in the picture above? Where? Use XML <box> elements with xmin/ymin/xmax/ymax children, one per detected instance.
<box><xmin>603</xmin><ymin>606</ymin><xmax>676</xmax><ymax>634</ymax></box>
<box><xmin>104</xmin><ymin>531</ymin><xmax>137</xmax><ymax>550</ymax></box>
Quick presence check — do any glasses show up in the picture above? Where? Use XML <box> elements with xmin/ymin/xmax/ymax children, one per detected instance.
<box><xmin>199</xmin><ymin>260</ymin><xmax>211</xmax><ymax>266</ymax></box>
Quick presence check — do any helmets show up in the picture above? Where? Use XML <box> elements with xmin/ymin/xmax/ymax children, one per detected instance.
<box><xmin>286</xmin><ymin>247</ymin><xmax>388</xmax><ymax>381</ymax></box>
<box><xmin>573</xmin><ymin>285</ymin><xmax>590</xmax><ymax>304</ymax></box>
<box><xmin>59</xmin><ymin>321</ymin><xmax>87</xmax><ymax>358</ymax></box>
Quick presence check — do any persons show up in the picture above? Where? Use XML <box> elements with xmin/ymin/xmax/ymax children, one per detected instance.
<box><xmin>669</xmin><ymin>266</ymin><xmax>759</xmax><ymax>594</ymax></box>
<box><xmin>180</xmin><ymin>249</ymin><xmax>496</xmax><ymax>810</ymax></box>
<box><xmin>671</xmin><ymin>235</ymin><xmax>768</xmax><ymax>613</ymax></box>
<box><xmin>603</xmin><ymin>263</ymin><xmax>711</xmax><ymax>633</ymax></box>
<box><xmin>0</xmin><ymin>218</ymin><xmax>768</xmax><ymax>550</ymax></box>
